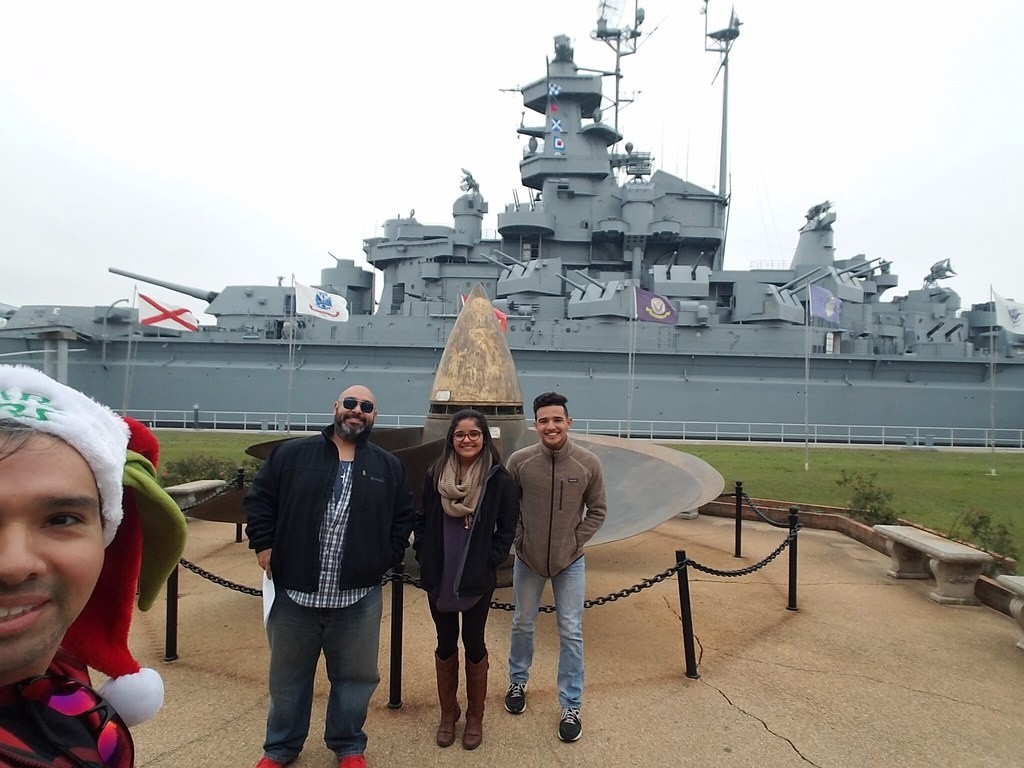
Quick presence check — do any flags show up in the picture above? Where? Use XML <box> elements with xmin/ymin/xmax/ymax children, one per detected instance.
<box><xmin>138</xmin><ymin>293</ymin><xmax>198</xmax><ymax>332</ymax></box>
<box><xmin>636</xmin><ymin>286</ymin><xmax>677</xmax><ymax>324</ymax></box>
<box><xmin>548</xmin><ymin>82</ymin><xmax>566</xmax><ymax>151</ymax></box>
<box><xmin>810</xmin><ymin>284</ymin><xmax>842</xmax><ymax>324</ymax></box>
<box><xmin>461</xmin><ymin>293</ymin><xmax>506</xmax><ymax>332</ymax></box>
<box><xmin>993</xmin><ymin>291</ymin><xmax>1024</xmax><ymax>334</ymax></box>
<box><xmin>296</xmin><ymin>282</ymin><xmax>349</xmax><ymax>322</ymax></box>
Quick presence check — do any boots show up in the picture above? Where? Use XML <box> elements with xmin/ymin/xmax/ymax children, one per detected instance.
<box><xmin>434</xmin><ymin>648</ymin><xmax>461</xmax><ymax>748</ymax></box>
<box><xmin>462</xmin><ymin>652</ymin><xmax>490</xmax><ymax>751</ymax></box>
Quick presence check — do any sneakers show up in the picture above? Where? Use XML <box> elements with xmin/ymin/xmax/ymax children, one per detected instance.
<box><xmin>557</xmin><ymin>706</ymin><xmax>583</xmax><ymax>742</ymax></box>
<box><xmin>254</xmin><ymin>757</ymin><xmax>286</xmax><ymax>768</ymax></box>
<box><xmin>504</xmin><ymin>680</ymin><xmax>528</xmax><ymax>714</ymax></box>
<box><xmin>338</xmin><ymin>754</ymin><xmax>366</xmax><ymax>768</ymax></box>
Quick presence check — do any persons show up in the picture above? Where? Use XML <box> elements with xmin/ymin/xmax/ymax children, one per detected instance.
<box><xmin>245</xmin><ymin>384</ymin><xmax>413</xmax><ymax>768</ymax></box>
<box><xmin>505</xmin><ymin>393</ymin><xmax>608</xmax><ymax>742</ymax></box>
<box><xmin>0</xmin><ymin>362</ymin><xmax>186</xmax><ymax>768</ymax></box>
<box><xmin>412</xmin><ymin>409</ymin><xmax>519</xmax><ymax>750</ymax></box>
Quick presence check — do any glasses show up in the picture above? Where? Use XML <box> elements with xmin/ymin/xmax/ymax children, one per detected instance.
<box><xmin>452</xmin><ymin>430</ymin><xmax>483</xmax><ymax>442</ymax></box>
<box><xmin>337</xmin><ymin>400</ymin><xmax>375</xmax><ymax>414</ymax></box>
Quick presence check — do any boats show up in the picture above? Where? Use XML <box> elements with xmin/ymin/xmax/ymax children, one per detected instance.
<box><xmin>2</xmin><ymin>4</ymin><xmax>1024</xmax><ymax>450</ymax></box>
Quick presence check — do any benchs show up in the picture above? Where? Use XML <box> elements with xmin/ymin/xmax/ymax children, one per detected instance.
<box><xmin>162</xmin><ymin>479</ymin><xmax>226</xmax><ymax>521</ymax></box>
<box><xmin>872</xmin><ymin>524</ymin><xmax>993</xmax><ymax>606</ymax></box>
<box><xmin>995</xmin><ymin>575</ymin><xmax>1024</xmax><ymax>651</ymax></box>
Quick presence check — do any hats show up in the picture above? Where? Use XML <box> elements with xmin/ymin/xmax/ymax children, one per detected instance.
<box><xmin>1</xmin><ymin>362</ymin><xmax>188</xmax><ymax>726</ymax></box>
